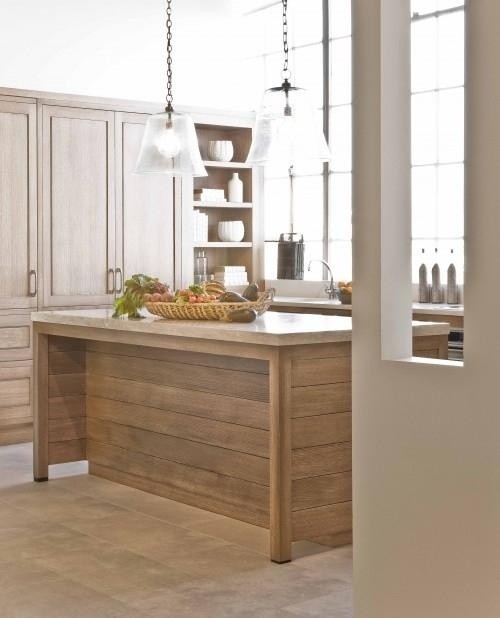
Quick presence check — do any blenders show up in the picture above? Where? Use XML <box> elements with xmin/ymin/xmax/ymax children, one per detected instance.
<box><xmin>276</xmin><ymin>233</ymin><xmax>305</xmax><ymax>280</ymax></box>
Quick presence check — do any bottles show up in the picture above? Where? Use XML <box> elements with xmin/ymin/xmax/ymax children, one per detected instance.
<box><xmin>414</xmin><ymin>247</ymin><xmax>461</xmax><ymax>305</ymax></box>
<box><xmin>227</xmin><ymin>172</ymin><xmax>244</xmax><ymax>202</ymax></box>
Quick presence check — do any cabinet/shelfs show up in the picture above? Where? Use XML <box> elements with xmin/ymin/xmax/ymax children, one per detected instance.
<box><xmin>0</xmin><ymin>86</ymin><xmax>261</xmax><ymax>446</ymax></box>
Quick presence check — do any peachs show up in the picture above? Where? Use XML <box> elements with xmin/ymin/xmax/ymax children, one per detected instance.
<box><xmin>142</xmin><ymin>292</ymin><xmax>172</xmax><ymax>303</ymax></box>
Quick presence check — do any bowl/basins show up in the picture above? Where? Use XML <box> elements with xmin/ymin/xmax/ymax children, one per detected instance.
<box><xmin>207</xmin><ymin>140</ymin><xmax>234</xmax><ymax>161</ymax></box>
<box><xmin>217</xmin><ymin>220</ymin><xmax>245</xmax><ymax>243</ymax></box>
<box><xmin>335</xmin><ymin>287</ymin><xmax>351</xmax><ymax>303</ymax></box>
<box><xmin>194</xmin><ymin>250</ymin><xmax>209</xmax><ymax>283</ymax></box>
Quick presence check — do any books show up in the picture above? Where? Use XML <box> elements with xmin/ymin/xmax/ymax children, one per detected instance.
<box><xmin>193</xmin><ymin>188</ymin><xmax>227</xmax><ymax>205</ymax></box>
<box><xmin>213</xmin><ymin>264</ymin><xmax>249</xmax><ymax>288</ymax></box>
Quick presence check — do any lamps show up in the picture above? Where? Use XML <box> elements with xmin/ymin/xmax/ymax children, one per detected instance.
<box><xmin>134</xmin><ymin>0</ymin><xmax>209</xmax><ymax>178</ymax></box>
<box><xmin>244</xmin><ymin>0</ymin><xmax>332</xmax><ymax>167</ymax></box>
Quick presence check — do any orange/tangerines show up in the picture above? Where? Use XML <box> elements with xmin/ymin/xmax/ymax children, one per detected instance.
<box><xmin>338</xmin><ymin>281</ymin><xmax>352</xmax><ymax>294</ymax></box>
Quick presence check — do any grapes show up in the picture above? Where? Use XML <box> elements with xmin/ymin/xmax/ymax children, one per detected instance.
<box><xmin>179</xmin><ymin>289</ymin><xmax>217</xmax><ymax>303</ymax></box>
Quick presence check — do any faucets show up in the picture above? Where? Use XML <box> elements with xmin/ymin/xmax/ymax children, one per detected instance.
<box><xmin>308</xmin><ymin>259</ymin><xmax>335</xmax><ymax>289</ymax></box>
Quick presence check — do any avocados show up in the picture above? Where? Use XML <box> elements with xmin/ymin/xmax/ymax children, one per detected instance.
<box><xmin>243</xmin><ymin>284</ymin><xmax>258</xmax><ymax>301</ymax></box>
<box><xmin>228</xmin><ymin>308</ymin><xmax>256</xmax><ymax>322</ymax></box>
<box><xmin>219</xmin><ymin>292</ymin><xmax>247</xmax><ymax>301</ymax></box>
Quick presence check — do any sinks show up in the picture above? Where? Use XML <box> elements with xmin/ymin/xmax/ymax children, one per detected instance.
<box><xmin>278</xmin><ymin>297</ymin><xmax>332</xmax><ymax>303</ymax></box>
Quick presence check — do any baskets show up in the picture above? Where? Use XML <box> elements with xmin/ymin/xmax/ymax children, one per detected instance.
<box><xmin>144</xmin><ymin>287</ymin><xmax>275</xmax><ymax>321</ymax></box>
